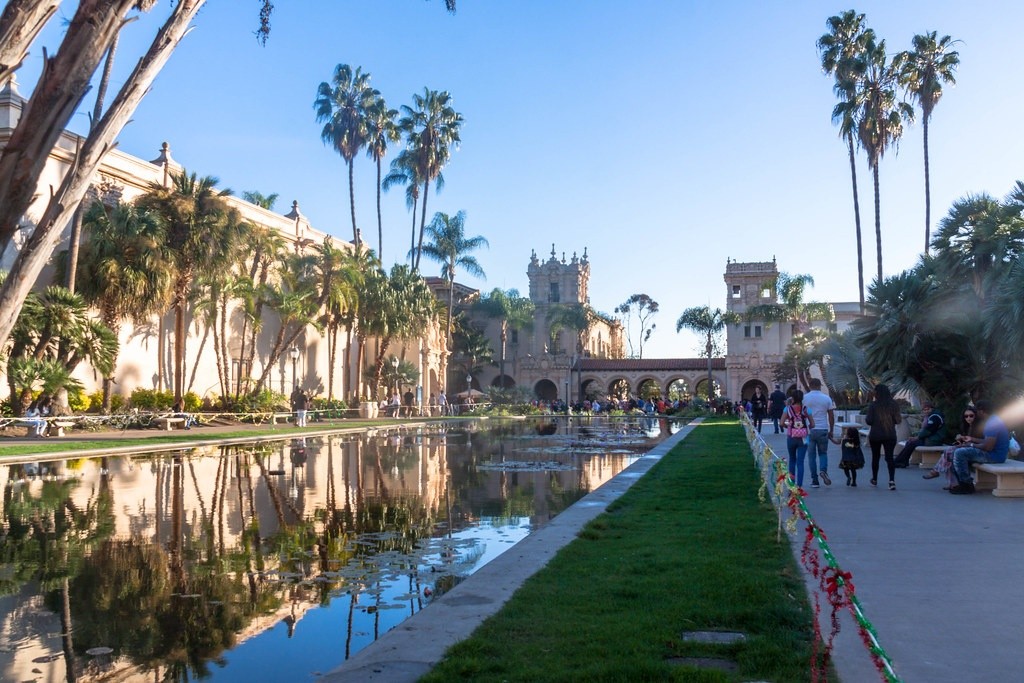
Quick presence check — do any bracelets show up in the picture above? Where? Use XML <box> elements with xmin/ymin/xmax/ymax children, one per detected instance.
<box><xmin>970</xmin><ymin>443</ymin><xmax>974</xmax><ymax>447</ymax></box>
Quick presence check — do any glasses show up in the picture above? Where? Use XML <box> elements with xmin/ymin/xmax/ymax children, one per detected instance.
<box><xmin>963</xmin><ymin>414</ymin><xmax>974</xmax><ymax>418</ymax></box>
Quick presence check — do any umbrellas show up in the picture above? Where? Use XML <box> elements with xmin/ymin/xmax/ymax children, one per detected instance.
<box><xmin>454</xmin><ymin>389</ymin><xmax>487</xmax><ymax>400</ymax></box>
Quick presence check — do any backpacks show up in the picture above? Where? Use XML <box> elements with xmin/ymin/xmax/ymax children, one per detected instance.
<box><xmin>788</xmin><ymin>404</ymin><xmax>807</xmax><ymax>437</ymax></box>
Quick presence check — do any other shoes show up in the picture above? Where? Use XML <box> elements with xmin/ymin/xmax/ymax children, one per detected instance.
<box><xmin>781</xmin><ymin>427</ymin><xmax>784</xmax><ymax>432</ymax></box>
<box><xmin>888</xmin><ymin>481</ymin><xmax>896</xmax><ymax>489</ymax></box>
<box><xmin>809</xmin><ymin>482</ymin><xmax>819</xmax><ymax>487</ymax></box>
<box><xmin>775</xmin><ymin>431</ymin><xmax>779</xmax><ymax>434</ymax></box>
<box><xmin>943</xmin><ymin>483</ymin><xmax>958</xmax><ymax>490</ymax></box>
<box><xmin>820</xmin><ymin>471</ymin><xmax>831</xmax><ymax>486</ymax></box>
<box><xmin>847</xmin><ymin>477</ymin><xmax>851</xmax><ymax>485</ymax></box>
<box><xmin>851</xmin><ymin>483</ymin><xmax>856</xmax><ymax>486</ymax></box>
<box><xmin>923</xmin><ymin>470</ymin><xmax>940</xmax><ymax>478</ymax></box>
<box><xmin>870</xmin><ymin>478</ymin><xmax>876</xmax><ymax>485</ymax></box>
<box><xmin>950</xmin><ymin>483</ymin><xmax>974</xmax><ymax>494</ymax></box>
<box><xmin>893</xmin><ymin>461</ymin><xmax>909</xmax><ymax>468</ymax></box>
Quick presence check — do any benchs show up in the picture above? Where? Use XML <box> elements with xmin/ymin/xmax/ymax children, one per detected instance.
<box><xmin>898</xmin><ymin>441</ymin><xmax>949</xmax><ymax>469</ymax></box>
<box><xmin>11</xmin><ymin>422</ymin><xmax>74</xmax><ymax>437</ymax></box>
<box><xmin>858</xmin><ymin>426</ymin><xmax>872</xmax><ymax>446</ymax></box>
<box><xmin>972</xmin><ymin>459</ymin><xmax>1024</xmax><ymax>497</ymax></box>
<box><xmin>270</xmin><ymin>413</ymin><xmax>294</xmax><ymax>424</ymax></box>
<box><xmin>153</xmin><ymin>418</ymin><xmax>188</xmax><ymax>430</ymax></box>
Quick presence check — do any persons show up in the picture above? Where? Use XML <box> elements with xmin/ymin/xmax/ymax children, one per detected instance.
<box><xmin>404</xmin><ymin>388</ymin><xmax>415</xmax><ymax>419</ymax></box>
<box><xmin>865</xmin><ymin>384</ymin><xmax>902</xmax><ymax>490</ymax></box>
<box><xmin>949</xmin><ymin>399</ymin><xmax>1009</xmax><ymax>495</ymax></box>
<box><xmin>26</xmin><ymin>401</ymin><xmax>48</xmax><ymax>438</ymax></box>
<box><xmin>922</xmin><ymin>406</ymin><xmax>985</xmax><ymax>490</ymax></box>
<box><xmin>780</xmin><ymin>390</ymin><xmax>815</xmax><ymax>488</ymax></box>
<box><xmin>750</xmin><ymin>385</ymin><xmax>767</xmax><ymax>434</ymax></box>
<box><xmin>768</xmin><ymin>385</ymin><xmax>787</xmax><ymax>434</ymax></box>
<box><xmin>801</xmin><ymin>378</ymin><xmax>836</xmax><ymax>488</ymax></box>
<box><xmin>464</xmin><ymin>395</ymin><xmax>474</xmax><ymax>410</ymax></box>
<box><xmin>392</xmin><ymin>389</ymin><xmax>401</xmax><ymax>419</ymax></box>
<box><xmin>531</xmin><ymin>394</ymin><xmax>674</xmax><ymax>415</ymax></box>
<box><xmin>172</xmin><ymin>399</ymin><xmax>203</xmax><ymax>430</ymax></box>
<box><xmin>291</xmin><ymin>385</ymin><xmax>301</xmax><ymax>424</ymax></box>
<box><xmin>438</xmin><ymin>390</ymin><xmax>446</xmax><ymax>417</ymax></box>
<box><xmin>429</xmin><ymin>393</ymin><xmax>436</xmax><ymax>416</ymax></box>
<box><xmin>38</xmin><ymin>396</ymin><xmax>63</xmax><ymax>437</ymax></box>
<box><xmin>292</xmin><ymin>389</ymin><xmax>308</xmax><ymax>427</ymax></box>
<box><xmin>380</xmin><ymin>396</ymin><xmax>392</xmax><ymax>417</ymax></box>
<box><xmin>673</xmin><ymin>396</ymin><xmax>753</xmax><ymax>419</ymax></box>
<box><xmin>894</xmin><ymin>400</ymin><xmax>946</xmax><ymax>468</ymax></box>
<box><xmin>828</xmin><ymin>427</ymin><xmax>865</xmax><ymax>486</ymax></box>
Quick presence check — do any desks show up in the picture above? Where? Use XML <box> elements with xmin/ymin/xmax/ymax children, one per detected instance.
<box><xmin>835</xmin><ymin>422</ymin><xmax>863</xmax><ymax>439</ymax></box>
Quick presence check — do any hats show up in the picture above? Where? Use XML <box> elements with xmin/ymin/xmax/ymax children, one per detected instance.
<box><xmin>922</xmin><ymin>400</ymin><xmax>934</xmax><ymax>410</ymax></box>
